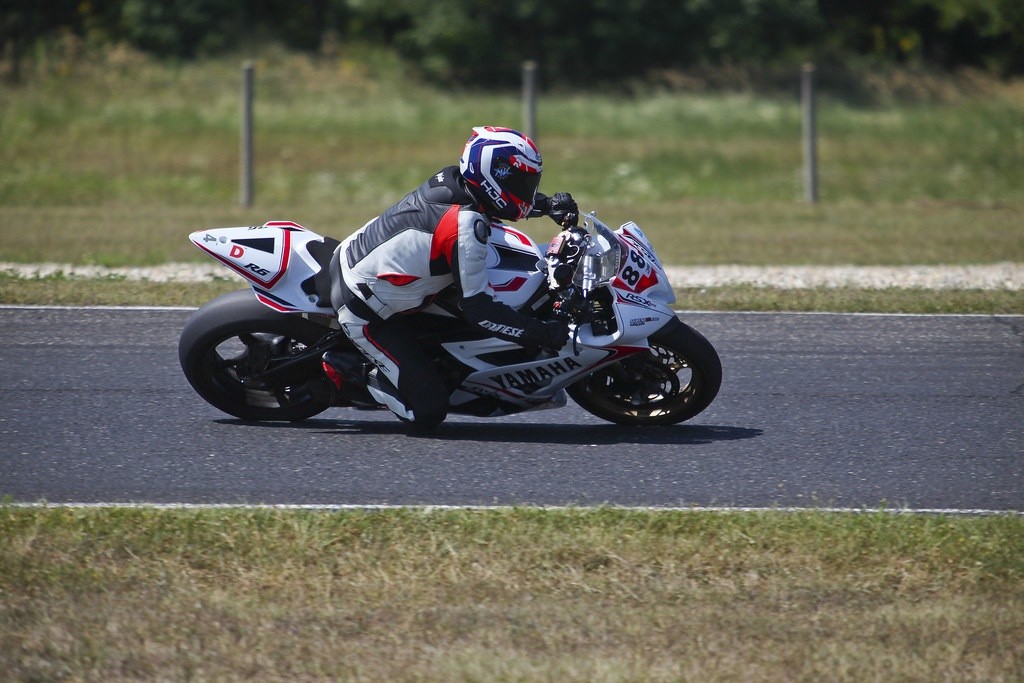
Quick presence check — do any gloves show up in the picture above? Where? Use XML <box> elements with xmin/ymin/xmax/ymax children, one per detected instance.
<box><xmin>521</xmin><ymin>318</ymin><xmax>571</xmax><ymax>352</ymax></box>
<box><xmin>543</xmin><ymin>193</ymin><xmax>578</xmax><ymax>226</ymax></box>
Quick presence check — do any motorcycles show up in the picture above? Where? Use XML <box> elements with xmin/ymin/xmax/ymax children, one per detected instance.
<box><xmin>177</xmin><ymin>193</ymin><xmax>724</xmax><ymax>428</ymax></box>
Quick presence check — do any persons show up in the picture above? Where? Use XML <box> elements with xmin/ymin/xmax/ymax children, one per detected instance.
<box><xmin>322</xmin><ymin>124</ymin><xmax>579</xmax><ymax>423</ymax></box>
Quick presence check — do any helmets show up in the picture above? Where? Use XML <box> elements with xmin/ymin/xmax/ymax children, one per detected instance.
<box><xmin>461</xmin><ymin>126</ymin><xmax>543</xmax><ymax>221</ymax></box>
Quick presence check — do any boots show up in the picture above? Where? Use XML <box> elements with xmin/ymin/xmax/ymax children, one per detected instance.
<box><xmin>320</xmin><ymin>352</ymin><xmax>380</xmax><ymax>410</ymax></box>
